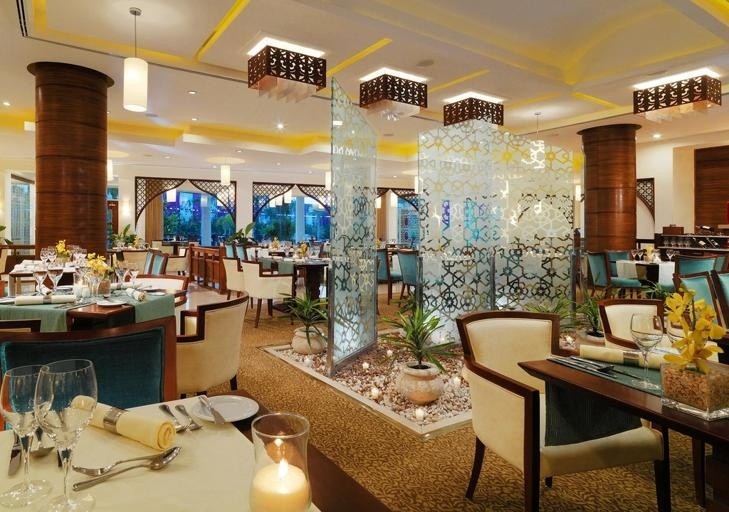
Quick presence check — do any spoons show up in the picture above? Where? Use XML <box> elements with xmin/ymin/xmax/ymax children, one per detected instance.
<box><xmin>27</xmin><ymin>427</ymin><xmax>56</xmax><ymax>460</ymax></box>
<box><xmin>551</xmin><ymin>352</ymin><xmax>614</xmax><ymax>374</ymax></box>
<box><xmin>70</xmin><ymin>446</ymin><xmax>183</xmax><ymax>493</ymax></box>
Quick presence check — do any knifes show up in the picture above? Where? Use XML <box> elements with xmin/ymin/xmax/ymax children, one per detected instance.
<box><xmin>7</xmin><ymin>431</ymin><xmax>24</xmax><ymax>477</ymax></box>
<box><xmin>198</xmin><ymin>395</ymin><xmax>227</xmax><ymax>428</ymax></box>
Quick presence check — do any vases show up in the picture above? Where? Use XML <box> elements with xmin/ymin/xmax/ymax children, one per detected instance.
<box><xmin>659</xmin><ymin>359</ymin><xmax>729</xmax><ymax>412</ymax></box>
<box><xmin>83</xmin><ymin>274</ymin><xmax>103</xmax><ymax>299</ymax></box>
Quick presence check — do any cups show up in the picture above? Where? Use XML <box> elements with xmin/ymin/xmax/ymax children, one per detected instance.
<box><xmin>46</xmin><ymin>262</ymin><xmax>64</xmax><ymax>297</ymax></box>
<box><xmin>249</xmin><ymin>412</ymin><xmax>311</xmax><ymax>512</ymax></box>
<box><xmin>73</xmin><ymin>260</ymin><xmax>103</xmax><ymax>300</ymax></box>
<box><xmin>667</xmin><ymin>250</ymin><xmax>675</xmax><ymax>260</ymax></box>
<box><xmin>637</xmin><ymin>249</ymin><xmax>644</xmax><ymax>260</ymax></box>
<box><xmin>630</xmin><ymin>312</ymin><xmax>663</xmax><ymax>392</ymax></box>
<box><xmin>127</xmin><ymin>261</ymin><xmax>139</xmax><ymax>291</ymax></box>
<box><xmin>667</xmin><ymin>310</ymin><xmax>691</xmax><ymax>357</ymax></box>
<box><xmin>67</xmin><ymin>243</ymin><xmax>87</xmax><ymax>267</ymax></box>
<box><xmin>40</xmin><ymin>245</ymin><xmax>49</xmax><ymax>265</ymax></box>
<box><xmin>0</xmin><ymin>363</ymin><xmax>53</xmax><ymax>508</ymax></box>
<box><xmin>32</xmin><ymin>263</ymin><xmax>47</xmax><ymax>297</ymax></box>
<box><xmin>663</xmin><ymin>236</ymin><xmax>691</xmax><ymax>248</ymax></box>
<box><xmin>632</xmin><ymin>249</ymin><xmax>638</xmax><ymax>261</ymax></box>
<box><xmin>114</xmin><ymin>260</ymin><xmax>129</xmax><ymax>293</ymax></box>
<box><xmin>32</xmin><ymin>357</ymin><xmax>99</xmax><ymax>512</ymax></box>
<box><xmin>47</xmin><ymin>244</ymin><xmax>57</xmax><ymax>264</ymax></box>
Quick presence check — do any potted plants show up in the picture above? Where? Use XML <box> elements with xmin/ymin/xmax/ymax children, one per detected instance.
<box><xmin>235</xmin><ymin>220</ymin><xmax>256</xmax><ymax>259</ymax></box>
<box><xmin>225</xmin><ymin>234</ymin><xmax>236</xmax><ymax>259</ymax></box>
<box><xmin>279</xmin><ymin>290</ymin><xmax>329</xmax><ymax>356</ymax></box>
<box><xmin>378</xmin><ymin>308</ymin><xmax>455</xmax><ymax>405</ymax></box>
<box><xmin>578</xmin><ymin>291</ymin><xmax>608</xmax><ymax>347</ymax></box>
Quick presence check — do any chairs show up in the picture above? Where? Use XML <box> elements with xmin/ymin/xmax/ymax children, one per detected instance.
<box><xmin>608</xmin><ymin>252</ymin><xmax>633</xmax><ymax>279</ymax></box>
<box><xmin>240</xmin><ymin>259</ymin><xmax>296</xmax><ymax>329</ymax></box>
<box><xmin>671</xmin><ymin>269</ymin><xmax>723</xmax><ymax>329</ymax></box>
<box><xmin>234</xmin><ymin>244</ymin><xmax>246</xmax><ymax>261</ymax></box>
<box><xmin>397</xmin><ymin>246</ymin><xmax>424</xmax><ymax>309</ymax></box>
<box><xmin>586</xmin><ymin>249</ymin><xmax>642</xmax><ymax>304</ymax></box>
<box><xmin>174</xmin><ymin>289</ymin><xmax>250</xmax><ymax>403</ymax></box>
<box><xmin>708</xmin><ymin>269</ymin><xmax>729</xmax><ymax>329</ymax></box>
<box><xmin>596</xmin><ymin>298</ymin><xmax>666</xmax><ymax>352</ymax></box>
<box><xmin>672</xmin><ymin>254</ymin><xmax>718</xmax><ymax>277</ymax></box>
<box><xmin>222</xmin><ymin>256</ymin><xmax>248</xmax><ymax>301</ymax></box>
<box><xmin>151</xmin><ymin>240</ymin><xmax>162</xmax><ymax>251</ymax></box>
<box><xmin>223</xmin><ymin>242</ymin><xmax>235</xmax><ymax>259</ymax></box>
<box><xmin>713</xmin><ymin>254</ymin><xmax>727</xmax><ymax>274</ymax></box>
<box><xmin>455</xmin><ymin>310</ymin><xmax>675</xmax><ymax>512</ymax></box>
<box><xmin>124</xmin><ymin>272</ymin><xmax>191</xmax><ymax>303</ymax></box>
<box><xmin>143</xmin><ymin>248</ymin><xmax>161</xmax><ymax>276</ymax></box>
<box><xmin>149</xmin><ymin>252</ymin><xmax>169</xmax><ymax>278</ymax></box>
<box><xmin>0</xmin><ymin>313</ymin><xmax>178</xmax><ymax>430</ymax></box>
<box><xmin>376</xmin><ymin>248</ymin><xmax>402</xmax><ymax>307</ymax></box>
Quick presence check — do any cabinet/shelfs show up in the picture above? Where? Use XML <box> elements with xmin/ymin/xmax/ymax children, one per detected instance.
<box><xmin>654</xmin><ymin>233</ymin><xmax>729</xmax><ymax>257</ymax></box>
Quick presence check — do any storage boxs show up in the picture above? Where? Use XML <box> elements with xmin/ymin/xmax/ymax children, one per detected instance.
<box><xmin>662</xmin><ymin>224</ymin><xmax>684</xmax><ymax>236</ymax></box>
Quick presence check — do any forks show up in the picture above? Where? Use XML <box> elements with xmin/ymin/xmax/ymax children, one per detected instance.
<box><xmin>157</xmin><ymin>404</ymin><xmax>189</xmax><ymax>433</ymax></box>
<box><xmin>71</xmin><ymin>452</ymin><xmax>160</xmax><ymax>477</ymax></box>
<box><xmin>174</xmin><ymin>404</ymin><xmax>204</xmax><ymax>431</ymax></box>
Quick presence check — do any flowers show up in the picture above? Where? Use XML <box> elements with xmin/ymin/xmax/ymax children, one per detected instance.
<box><xmin>662</xmin><ymin>288</ymin><xmax>729</xmax><ymax>373</ymax></box>
<box><xmin>81</xmin><ymin>250</ymin><xmax>113</xmax><ymax>281</ymax></box>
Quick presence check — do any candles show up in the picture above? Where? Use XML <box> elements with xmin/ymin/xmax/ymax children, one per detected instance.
<box><xmin>254</xmin><ymin>460</ymin><xmax>307</xmax><ymax>508</ymax></box>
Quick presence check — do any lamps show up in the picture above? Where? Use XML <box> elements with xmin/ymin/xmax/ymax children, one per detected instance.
<box><xmin>219</xmin><ymin>163</ymin><xmax>230</xmax><ymax>187</ymax></box>
<box><xmin>631</xmin><ymin>70</ymin><xmax>723</xmax><ymax>123</ymax></box>
<box><xmin>121</xmin><ymin>55</ymin><xmax>149</xmax><ymax>115</ymax></box>
<box><xmin>358</xmin><ymin>68</ymin><xmax>427</xmax><ymax>125</ymax></box>
<box><xmin>413</xmin><ymin>175</ymin><xmax>425</xmax><ymax>196</ymax></box>
<box><xmin>105</xmin><ymin>158</ymin><xmax>114</xmax><ymax>183</ymax></box>
<box><xmin>324</xmin><ymin>170</ymin><xmax>334</xmax><ymax>191</ymax></box>
<box><xmin>442</xmin><ymin>91</ymin><xmax>505</xmax><ymax>130</ymax></box>
<box><xmin>246</xmin><ymin>35</ymin><xmax>328</xmax><ymax>103</ymax></box>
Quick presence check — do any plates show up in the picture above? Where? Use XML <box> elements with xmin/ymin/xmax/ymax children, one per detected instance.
<box><xmin>1</xmin><ymin>297</ymin><xmax>16</xmax><ymax>304</ymax></box>
<box><xmin>190</xmin><ymin>394</ymin><xmax>260</xmax><ymax>423</ymax></box>
<box><xmin>95</xmin><ymin>299</ymin><xmax>127</xmax><ymax>307</ymax></box>
